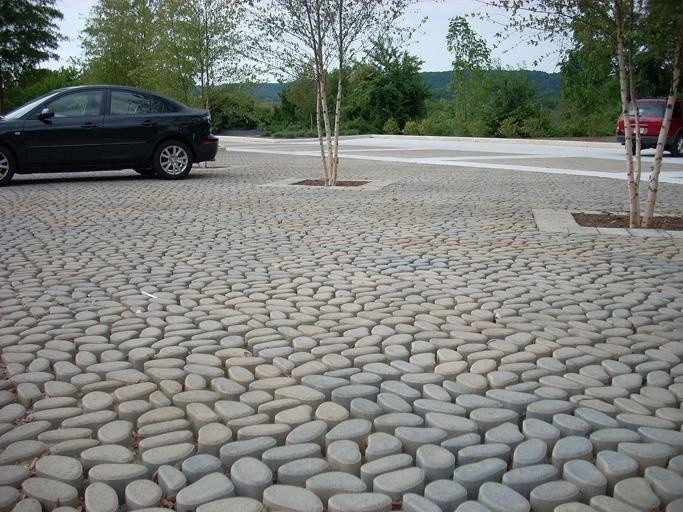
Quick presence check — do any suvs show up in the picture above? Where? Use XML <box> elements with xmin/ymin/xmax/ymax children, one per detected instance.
<box><xmin>615</xmin><ymin>98</ymin><xmax>683</xmax><ymax>155</ymax></box>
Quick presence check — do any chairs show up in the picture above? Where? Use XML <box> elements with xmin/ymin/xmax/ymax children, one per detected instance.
<box><xmin>81</xmin><ymin>98</ymin><xmax>99</xmax><ymax>116</ymax></box>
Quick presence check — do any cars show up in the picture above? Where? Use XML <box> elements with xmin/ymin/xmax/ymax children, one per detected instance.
<box><xmin>0</xmin><ymin>85</ymin><xmax>220</xmax><ymax>184</ymax></box>
